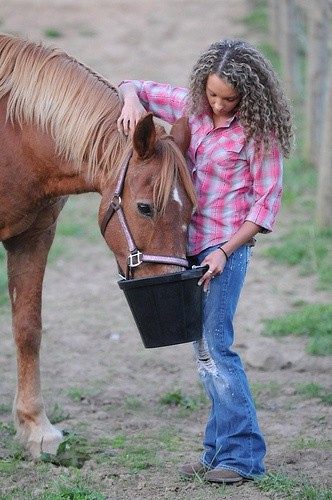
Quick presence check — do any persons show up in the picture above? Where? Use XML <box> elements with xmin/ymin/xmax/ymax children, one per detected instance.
<box><xmin>116</xmin><ymin>39</ymin><xmax>292</xmax><ymax>482</ymax></box>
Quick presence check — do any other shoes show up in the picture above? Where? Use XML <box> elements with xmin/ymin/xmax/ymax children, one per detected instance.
<box><xmin>204</xmin><ymin>468</ymin><xmax>244</xmax><ymax>482</ymax></box>
<box><xmin>178</xmin><ymin>462</ymin><xmax>211</xmax><ymax>478</ymax></box>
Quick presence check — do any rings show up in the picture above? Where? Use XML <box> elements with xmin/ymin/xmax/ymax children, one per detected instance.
<box><xmin>208</xmin><ymin>270</ymin><xmax>212</xmax><ymax>274</ymax></box>
<box><xmin>212</xmin><ymin>276</ymin><xmax>214</xmax><ymax>278</ymax></box>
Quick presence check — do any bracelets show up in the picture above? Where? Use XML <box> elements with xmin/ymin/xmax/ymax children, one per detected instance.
<box><xmin>217</xmin><ymin>246</ymin><xmax>228</xmax><ymax>262</ymax></box>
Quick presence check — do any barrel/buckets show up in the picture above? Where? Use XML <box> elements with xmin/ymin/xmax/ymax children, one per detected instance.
<box><xmin>117</xmin><ymin>265</ymin><xmax>209</xmax><ymax>349</ymax></box>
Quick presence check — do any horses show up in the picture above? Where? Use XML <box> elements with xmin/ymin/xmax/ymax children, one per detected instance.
<box><xmin>0</xmin><ymin>34</ymin><xmax>202</xmax><ymax>462</ymax></box>
